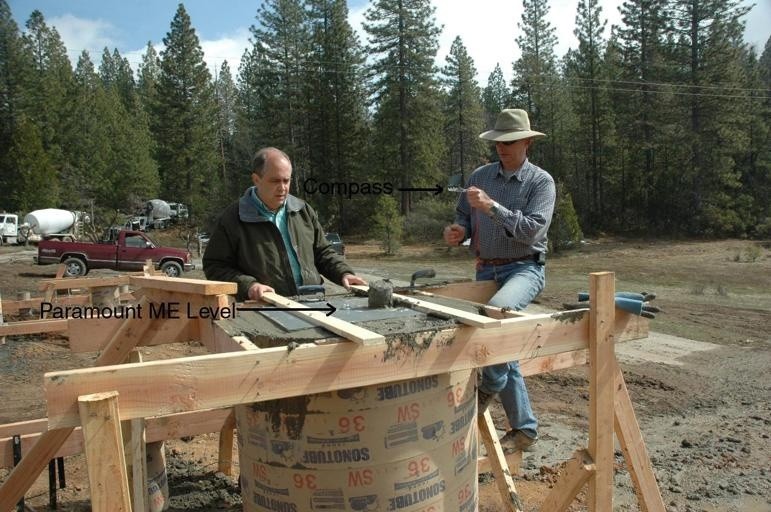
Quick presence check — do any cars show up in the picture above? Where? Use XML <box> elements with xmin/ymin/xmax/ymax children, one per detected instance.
<box><xmin>323</xmin><ymin>232</ymin><xmax>344</xmax><ymax>255</ymax></box>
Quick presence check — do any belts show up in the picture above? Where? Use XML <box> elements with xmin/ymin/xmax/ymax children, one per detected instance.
<box><xmin>478</xmin><ymin>253</ymin><xmax>537</xmax><ymax>266</ymax></box>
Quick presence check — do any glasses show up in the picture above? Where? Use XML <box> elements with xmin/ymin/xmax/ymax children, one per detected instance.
<box><xmin>495</xmin><ymin>140</ymin><xmax>517</xmax><ymax>145</ymax></box>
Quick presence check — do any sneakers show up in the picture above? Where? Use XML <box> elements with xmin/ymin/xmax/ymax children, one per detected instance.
<box><xmin>478</xmin><ymin>385</ymin><xmax>497</xmax><ymax>418</ymax></box>
<box><xmin>483</xmin><ymin>430</ymin><xmax>538</xmax><ymax>457</ymax></box>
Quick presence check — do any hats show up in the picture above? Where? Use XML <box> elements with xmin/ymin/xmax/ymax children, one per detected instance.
<box><xmin>479</xmin><ymin>108</ymin><xmax>548</xmax><ymax>142</ymax></box>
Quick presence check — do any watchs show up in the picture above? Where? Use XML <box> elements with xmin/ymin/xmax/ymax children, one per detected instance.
<box><xmin>488</xmin><ymin>202</ymin><xmax>499</xmax><ymax>217</ymax></box>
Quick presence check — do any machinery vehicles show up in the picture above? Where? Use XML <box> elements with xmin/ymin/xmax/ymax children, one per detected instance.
<box><xmin>127</xmin><ymin>197</ymin><xmax>189</xmax><ymax>232</ymax></box>
<box><xmin>0</xmin><ymin>207</ymin><xmax>82</xmax><ymax>243</ymax></box>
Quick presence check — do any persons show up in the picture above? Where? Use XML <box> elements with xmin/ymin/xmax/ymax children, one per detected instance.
<box><xmin>202</xmin><ymin>147</ymin><xmax>368</xmax><ymax>302</ymax></box>
<box><xmin>442</xmin><ymin>110</ymin><xmax>556</xmax><ymax>457</ymax></box>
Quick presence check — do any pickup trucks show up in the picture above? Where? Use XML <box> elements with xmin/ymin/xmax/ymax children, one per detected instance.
<box><xmin>38</xmin><ymin>230</ymin><xmax>195</xmax><ymax>278</ymax></box>
